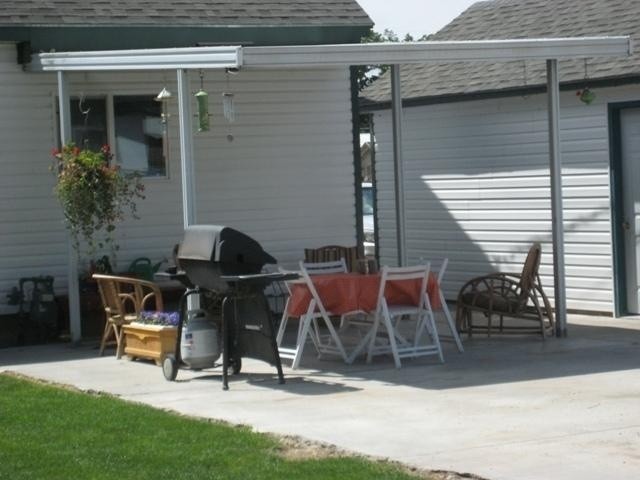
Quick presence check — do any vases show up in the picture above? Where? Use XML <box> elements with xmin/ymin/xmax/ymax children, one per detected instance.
<box><xmin>122</xmin><ymin>323</ymin><xmax>178</xmax><ymax>366</ymax></box>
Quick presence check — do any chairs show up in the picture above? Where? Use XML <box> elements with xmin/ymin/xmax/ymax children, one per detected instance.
<box><xmin>92</xmin><ymin>274</ymin><xmax>164</xmax><ymax>359</ymax></box>
<box><xmin>456</xmin><ymin>243</ymin><xmax>555</xmax><ymax>340</ymax></box>
<box><xmin>276</xmin><ymin>243</ymin><xmax>464</xmax><ymax>370</ymax></box>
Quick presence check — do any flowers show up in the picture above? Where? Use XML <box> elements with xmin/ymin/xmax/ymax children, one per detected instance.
<box><xmin>136</xmin><ymin>311</ymin><xmax>180</xmax><ymax>326</ymax></box>
